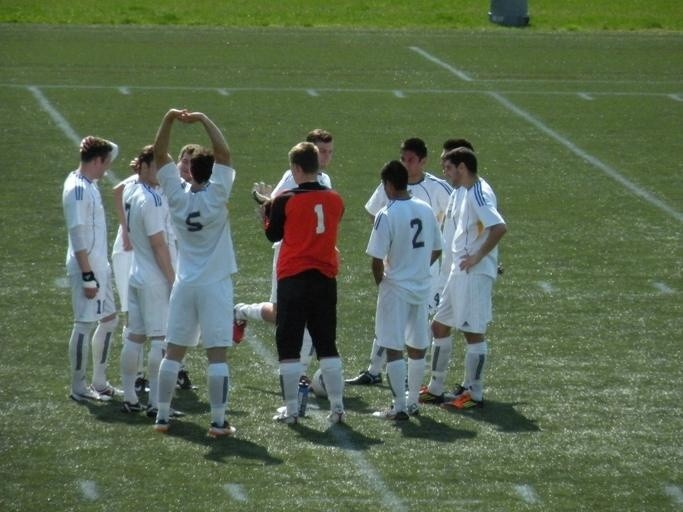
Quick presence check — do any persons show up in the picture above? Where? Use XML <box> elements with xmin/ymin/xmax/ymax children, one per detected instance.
<box><xmin>61</xmin><ymin>135</ymin><xmax>124</xmax><ymax>406</ymax></box>
<box><xmin>251</xmin><ymin>143</ymin><xmax>346</xmax><ymax>427</ymax></box>
<box><xmin>234</xmin><ymin>127</ymin><xmax>333</xmax><ymax>391</ymax></box>
<box><xmin>153</xmin><ymin>107</ymin><xmax>238</xmax><ymax>440</ymax></box>
<box><xmin>365</xmin><ymin>160</ymin><xmax>444</xmax><ymax>422</ymax></box>
<box><xmin>121</xmin><ymin>146</ymin><xmax>175</xmax><ymax>416</ymax></box>
<box><xmin>346</xmin><ymin>136</ymin><xmax>450</xmax><ymax>386</ymax></box>
<box><xmin>164</xmin><ymin>143</ymin><xmax>201</xmax><ymax>391</ymax></box>
<box><xmin>413</xmin><ymin>149</ymin><xmax>506</xmax><ymax>413</ymax></box>
<box><xmin>440</xmin><ymin>139</ymin><xmax>492</xmax><ymax>402</ymax></box>
<box><xmin>112</xmin><ymin>158</ymin><xmax>150</xmax><ymax>395</ymax></box>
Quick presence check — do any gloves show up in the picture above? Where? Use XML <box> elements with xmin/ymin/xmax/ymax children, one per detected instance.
<box><xmin>251</xmin><ymin>181</ymin><xmax>274</xmax><ymax>208</ymax></box>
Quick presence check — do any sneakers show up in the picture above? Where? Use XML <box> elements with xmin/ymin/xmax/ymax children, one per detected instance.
<box><xmin>343</xmin><ymin>371</ymin><xmax>385</xmax><ymax>386</ymax></box>
<box><xmin>296</xmin><ymin>377</ymin><xmax>313</xmax><ymax>418</ymax></box>
<box><xmin>407</xmin><ymin>404</ymin><xmax>421</xmax><ymax>416</ymax></box>
<box><xmin>68</xmin><ymin>386</ymin><xmax>112</xmax><ymax>407</ymax></box>
<box><xmin>175</xmin><ymin>370</ymin><xmax>192</xmax><ymax>391</ymax></box>
<box><xmin>438</xmin><ymin>392</ymin><xmax>484</xmax><ymax>410</ymax></box>
<box><xmin>144</xmin><ymin>404</ymin><xmax>185</xmax><ymax>419</ymax></box>
<box><xmin>232</xmin><ymin>302</ymin><xmax>245</xmax><ymax>343</ymax></box>
<box><xmin>153</xmin><ymin>419</ymin><xmax>169</xmax><ymax>433</ymax></box>
<box><xmin>135</xmin><ymin>379</ymin><xmax>153</xmax><ymax>393</ymax></box>
<box><xmin>272</xmin><ymin>410</ymin><xmax>299</xmax><ymax>426</ymax></box>
<box><xmin>325</xmin><ymin>408</ymin><xmax>348</xmax><ymax>424</ymax></box>
<box><xmin>371</xmin><ymin>406</ymin><xmax>410</xmax><ymax>420</ymax></box>
<box><xmin>405</xmin><ymin>384</ymin><xmax>428</xmax><ymax>395</ymax></box>
<box><xmin>419</xmin><ymin>390</ymin><xmax>444</xmax><ymax>403</ymax></box>
<box><xmin>444</xmin><ymin>385</ymin><xmax>469</xmax><ymax>399</ymax></box>
<box><xmin>207</xmin><ymin>421</ymin><xmax>237</xmax><ymax>437</ymax></box>
<box><xmin>88</xmin><ymin>384</ymin><xmax>124</xmax><ymax>397</ymax></box>
<box><xmin>120</xmin><ymin>401</ymin><xmax>148</xmax><ymax>413</ymax></box>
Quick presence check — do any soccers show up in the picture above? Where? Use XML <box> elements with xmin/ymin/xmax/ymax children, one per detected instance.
<box><xmin>312</xmin><ymin>369</ymin><xmax>345</xmax><ymax>396</ymax></box>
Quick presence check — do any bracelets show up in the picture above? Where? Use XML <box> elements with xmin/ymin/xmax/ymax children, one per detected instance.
<box><xmin>80</xmin><ymin>271</ymin><xmax>95</xmax><ymax>282</ymax></box>
